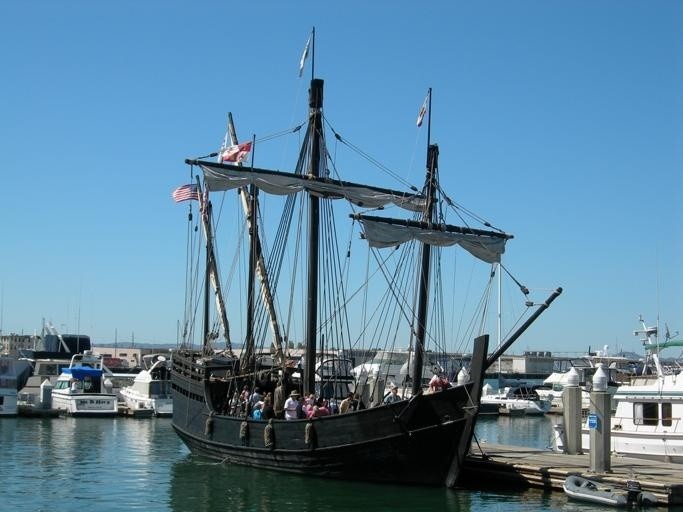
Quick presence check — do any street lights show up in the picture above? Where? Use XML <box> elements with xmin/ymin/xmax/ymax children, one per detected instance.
<box><xmin>589</xmin><ymin>365</ymin><xmax>612</xmax><ymax>475</ymax></box>
<box><xmin>561</xmin><ymin>366</ymin><xmax>584</xmax><ymax>456</ymax></box>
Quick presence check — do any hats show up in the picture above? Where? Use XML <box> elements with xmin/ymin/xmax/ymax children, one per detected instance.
<box><xmin>290</xmin><ymin>390</ymin><xmax>300</xmax><ymax>396</ymax></box>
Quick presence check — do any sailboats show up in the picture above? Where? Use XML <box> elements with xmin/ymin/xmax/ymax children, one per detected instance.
<box><xmin>167</xmin><ymin>20</ymin><xmax>562</xmax><ymax>489</ymax></box>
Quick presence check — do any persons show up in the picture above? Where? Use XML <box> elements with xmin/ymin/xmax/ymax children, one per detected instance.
<box><xmin>429</xmin><ymin>365</ymin><xmax>449</xmax><ymax>394</ymax></box>
<box><xmin>228</xmin><ymin>385</ymin><xmax>367</xmax><ymax>422</ymax></box>
<box><xmin>383</xmin><ymin>385</ymin><xmax>401</xmax><ymax>405</ymax></box>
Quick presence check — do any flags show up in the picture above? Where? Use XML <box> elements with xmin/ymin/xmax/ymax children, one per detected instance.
<box><xmin>172</xmin><ymin>183</ymin><xmax>203</xmax><ymax>203</ymax></box>
<box><xmin>416</xmin><ymin>94</ymin><xmax>428</xmax><ymax>128</ymax></box>
<box><xmin>222</xmin><ymin>141</ymin><xmax>255</xmax><ymax>164</ymax></box>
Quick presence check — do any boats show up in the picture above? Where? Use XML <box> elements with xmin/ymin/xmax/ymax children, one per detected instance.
<box><xmin>0</xmin><ymin>355</ymin><xmax>33</xmax><ymax>420</ymax></box>
<box><xmin>120</xmin><ymin>354</ymin><xmax>176</xmax><ymax>417</ymax></box>
<box><xmin>277</xmin><ymin>346</ymin><xmax>630</xmax><ymax>416</ymax></box>
<box><xmin>50</xmin><ymin>349</ymin><xmax>119</xmax><ymax>417</ymax></box>
<box><xmin>553</xmin><ymin>314</ymin><xmax>682</xmax><ymax>465</ymax></box>
<box><xmin>563</xmin><ymin>475</ymin><xmax>657</xmax><ymax>507</ymax></box>
